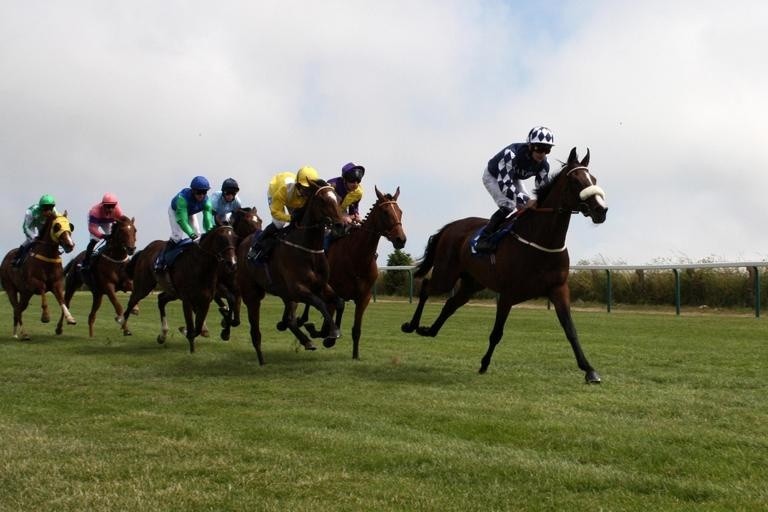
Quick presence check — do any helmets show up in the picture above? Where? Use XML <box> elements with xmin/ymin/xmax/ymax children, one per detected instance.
<box><xmin>342</xmin><ymin>162</ymin><xmax>365</xmax><ymax>178</ymax></box>
<box><xmin>39</xmin><ymin>194</ymin><xmax>55</xmax><ymax>207</ymax></box>
<box><xmin>190</xmin><ymin>176</ymin><xmax>211</xmax><ymax>190</ymax></box>
<box><xmin>526</xmin><ymin>126</ymin><xmax>555</xmax><ymax>146</ymax></box>
<box><xmin>222</xmin><ymin>178</ymin><xmax>239</xmax><ymax>192</ymax></box>
<box><xmin>298</xmin><ymin>165</ymin><xmax>318</xmax><ymax>186</ymax></box>
<box><xmin>102</xmin><ymin>192</ymin><xmax>117</xmax><ymax>205</ymax></box>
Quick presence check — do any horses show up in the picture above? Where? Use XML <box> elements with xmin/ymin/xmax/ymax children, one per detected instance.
<box><xmin>400</xmin><ymin>145</ymin><xmax>610</xmax><ymax>387</ymax></box>
<box><xmin>179</xmin><ymin>205</ymin><xmax>263</xmax><ymax>341</ymax></box>
<box><xmin>276</xmin><ymin>185</ymin><xmax>406</xmax><ymax>359</ymax></box>
<box><xmin>0</xmin><ymin>209</ymin><xmax>77</xmax><ymax>343</ymax></box>
<box><xmin>54</xmin><ymin>215</ymin><xmax>140</xmax><ymax>336</ymax></box>
<box><xmin>121</xmin><ymin>214</ymin><xmax>239</xmax><ymax>354</ymax></box>
<box><xmin>240</xmin><ymin>176</ymin><xmax>350</xmax><ymax>366</ymax></box>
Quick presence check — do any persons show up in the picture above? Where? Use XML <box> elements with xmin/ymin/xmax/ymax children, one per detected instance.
<box><xmin>252</xmin><ymin>166</ymin><xmax>321</xmax><ymax>253</ymax></box>
<box><xmin>152</xmin><ymin>175</ymin><xmax>213</xmax><ymax>273</ymax></box>
<box><xmin>10</xmin><ymin>193</ymin><xmax>63</xmax><ymax>267</ymax></box>
<box><xmin>469</xmin><ymin>126</ymin><xmax>556</xmax><ymax>253</ymax></box>
<box><xmin>75</xmin><ymin>193</ymin><xmax>122</xmax><ymax>271</ymax></box>
<box><xmin>210</xmin><ymin>178</ymin><xmax>243</xmax><ymax>226</ymax></box>
<box><xmin>326</xmin><ymin>161</ymin><xmax>366</xmax><ymax>225</ymax></box>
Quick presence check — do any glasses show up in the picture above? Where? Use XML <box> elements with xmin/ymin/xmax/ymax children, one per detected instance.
<box><xmin>42</xmin><ymin>208</ymin><xmax>53</xmax><ymax>210</ymax></box>
<box><xmin>105</xmin><ymin>205</ymin><xmax>115</xmax><ymax>208</ymax></box>
<box><xmin>193</xmin><ymin>191</ymin><xmax>207</xmax><ymax>195</ymax></box>
<box><xmin>531</xmin><ymin>143</ymin><xmax>551</xmax><ymax>154</ymax></box>
<box><xmin>224</xmin><ymin>192</ymin><xmax>236</xmax><ymax>196</ymax></box>
<box><xmin>346</xmin><ymin>178</ymin><xmax>362</xmax><ymax>182</ymax></box>
